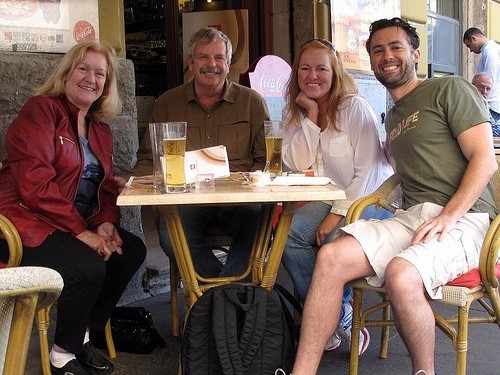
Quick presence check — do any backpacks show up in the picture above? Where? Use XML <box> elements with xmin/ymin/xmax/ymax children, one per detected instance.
<box><xmin>180</xmin><ymin>283</ymin><xmax>303</xmax><ymax>375</ymax></box>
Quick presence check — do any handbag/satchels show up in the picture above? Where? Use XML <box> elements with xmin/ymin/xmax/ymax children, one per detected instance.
<box><xmin>89</xmin><ymin>306</ymin><xmax>168</xmax><ymax>355</ymax></box>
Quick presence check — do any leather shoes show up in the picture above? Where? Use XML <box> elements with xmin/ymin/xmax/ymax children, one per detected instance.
<box><xmin>48</xmin><ymin>349</ymin><xmax>90</xmax><ymax>375</ymax></box>
<box><xmin>76</xmin><ymin>340</ymin><xmax>114</xmax><ymax>375</ymax></box>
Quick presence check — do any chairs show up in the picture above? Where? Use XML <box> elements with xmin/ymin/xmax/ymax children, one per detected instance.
<box><xmin>0</xmin><ymin>266</ymin><xmax>63</xmax><ymax>375</ymax></box>
<box><xmin>0</xmin><ymin>214</ymin><xmax>117</xmax><ymax>375</ymax></box>
<box><xmin>346</xmin><ymin>146</ymin><xmax>500</xmax><ymax>375</ymax></box>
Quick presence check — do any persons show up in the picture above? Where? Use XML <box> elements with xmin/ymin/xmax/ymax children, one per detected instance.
<box><xmin>0</xmin><ymin>42</ymin><xmax>147</xmax><ymax>375</ymax></box>
<box><xmin>281</xmin><ymin>38</ymin><xmax>402</xmax><ymax>358</ymax></box>
<box><xmin>275</xmin><ymin>17</ymin><xmax>500</xmax><ymax>375</ymax></box>
<box><xmin>471</xmin><ymin>72</ymin><xmax>500</xmax><ymax>137</ymax></box>
<box><xmin>128</xmin><ymin>27</ymin><xmax>274</xmax><ymax>285</ymax></box>
<box><xmin>463</xmin><ymin>28</ymin><xmax>500</xmax><ymax>123</ymax></box>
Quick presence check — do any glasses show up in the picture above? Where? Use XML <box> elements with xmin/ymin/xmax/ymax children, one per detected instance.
<box><xmin>472</xmin><ymin>82</ymin><xmax>491</xmax><ymax>91</ymax></box>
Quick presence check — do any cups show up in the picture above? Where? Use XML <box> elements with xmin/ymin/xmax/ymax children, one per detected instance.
<box><xmin>195</xmin><ymin>173</ymin><xmax>216</xmax><ymax>193</ymax></box>
<box><xmin>263</xmin><ymin>120</ymin><xmax>285</xmax><ymax>177</ymax></box>
<box><xmin>149</xmin><ymin>123</ymin><xmax>173</xmax><ymax>190</ymax></box>
<box><xmin>162</xmin><ymin>122</ymin><xmax>191</xmax><ymax>194</ymax></box>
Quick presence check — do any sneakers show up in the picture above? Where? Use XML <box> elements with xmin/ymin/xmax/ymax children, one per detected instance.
<box><xmin>335</xmin><ymin>298</ymin><xmax>371</xmax><ymax>360</ymax></box>
<box><xmin>325</xmin><ymin>331</ymin><xmax>341</xmax><ymax>351</ymax></box>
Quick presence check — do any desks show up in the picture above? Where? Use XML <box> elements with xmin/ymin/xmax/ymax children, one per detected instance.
<box><xmin>115</xmin><ymin>170</ymin><xmax>347</xmax><ymax>375</ymax></box>
